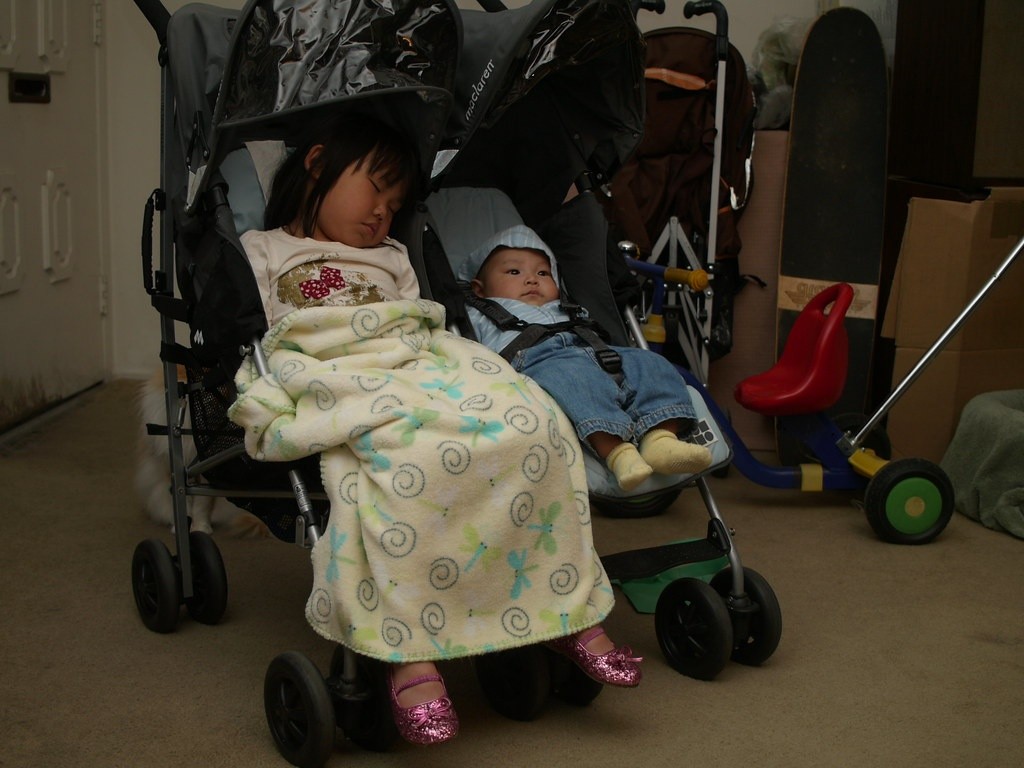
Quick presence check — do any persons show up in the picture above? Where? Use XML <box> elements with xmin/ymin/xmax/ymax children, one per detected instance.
<box><xmin>460</xmin><ymin>224</ymin><xmax>711</xmax><ymax>491</ymax></box>
<box><xmin>240</xmin><ymin>109</ymin><xmax>642</xmax><ymax>744</ymax></box>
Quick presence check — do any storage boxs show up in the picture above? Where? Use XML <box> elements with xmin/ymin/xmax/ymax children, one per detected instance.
<box><xmin>710</xmin><ymin>127</ymin><xmax>788</xmax><ymax>455</ymax></box>
<box><xmin>878</xmin><ymin>185</ymin><xmax>1024</xmax><ymax>462</ymax></box>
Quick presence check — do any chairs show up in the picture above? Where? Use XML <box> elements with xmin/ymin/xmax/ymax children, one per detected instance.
<box><xmin>734</xmin><ymin>279</ymin><xmax>855</xmax><ymax>415</ymax></box>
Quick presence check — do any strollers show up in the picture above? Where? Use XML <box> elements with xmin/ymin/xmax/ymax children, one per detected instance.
<box><xmin>129</xmin><ymin>1</ymin><xmax>606</xmax><ymax>768</ymax></box>
<box><xmin>421</xmin><ymin>0</ymin><xmax>783</xmax><ymax>682</ymax></box>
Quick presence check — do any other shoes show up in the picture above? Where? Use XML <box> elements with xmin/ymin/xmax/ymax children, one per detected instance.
<box><xmin>389</xmin><ymin>662</ymin><xmax>459</xmax><ymax>747</ymax></box>
<box><xmin>542</xmin><ymin>626</ymin><xmax>644</xmax><ymax>687</ymax></box>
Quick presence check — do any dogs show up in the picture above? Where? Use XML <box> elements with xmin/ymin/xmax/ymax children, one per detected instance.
<box><xmin>132</xmin><ymin>362</ymin><xmax>280</xmax><ymax>540</ymax></box>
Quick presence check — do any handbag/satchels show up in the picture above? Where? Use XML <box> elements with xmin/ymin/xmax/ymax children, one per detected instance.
<box><xmin>743</xmin><ymin>14</ymin><xmax>815</xmax><ymax>128</ymax></box>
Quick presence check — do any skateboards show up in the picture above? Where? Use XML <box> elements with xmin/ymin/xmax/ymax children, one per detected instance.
<box><xmin>773</xmin><ymin>5</ymin><xmax>895</xmax><ymax>479</ymax></box>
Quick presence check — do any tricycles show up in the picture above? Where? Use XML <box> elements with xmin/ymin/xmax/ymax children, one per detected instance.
<box><xmin>609</xmin><ymin>242</ymin><xmax>951</xmax><ymax>546</ymax></box>
